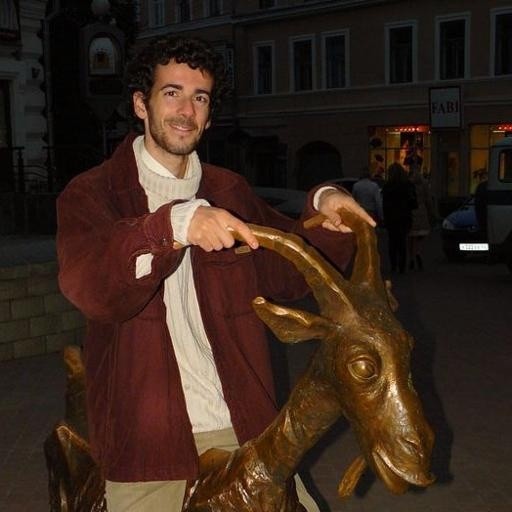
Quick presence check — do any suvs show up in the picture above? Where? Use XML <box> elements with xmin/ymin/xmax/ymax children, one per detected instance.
<box><xmin>475</xmin><ymin>135</ymin><xmax>512</xmax><ymax>273</ymax></box>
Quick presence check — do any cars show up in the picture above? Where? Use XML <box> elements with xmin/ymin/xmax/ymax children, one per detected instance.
<box><xmin>439</xmin><ymin>193</ymin><xmax>497</xmax><ymax>262</ymax></box>
<box><xmin>323</xmin><ymin>176</ymin><xmax>385</xmax><ymax>203</ymax></box>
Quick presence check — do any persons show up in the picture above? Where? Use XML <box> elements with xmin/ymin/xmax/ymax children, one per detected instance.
<box><xmin>55</xmin><ymin>36</ymin><xmax>378</xmax><ymax>512</ymax></box>
<box><xmin>380</xmin><ymin>162</ymin><xmax>418</xmax><ymax>276</ymax></box>
